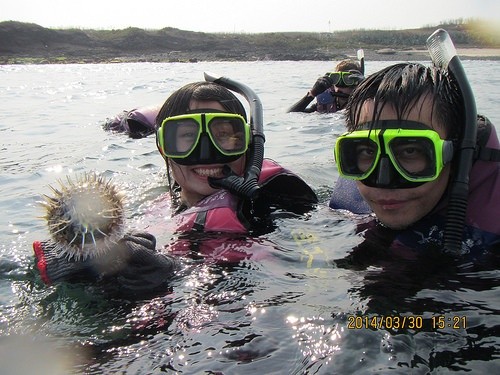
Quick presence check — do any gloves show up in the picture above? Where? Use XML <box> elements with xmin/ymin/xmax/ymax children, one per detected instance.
<box><xmin>348</xmin><ymin>70</ymin><xmax>365</xmax><ymax>81</ymax></box>
<box><xmin>310</xmin><ymin>73</ymin><xmax>332</xmax><ymax>96</ymax></box>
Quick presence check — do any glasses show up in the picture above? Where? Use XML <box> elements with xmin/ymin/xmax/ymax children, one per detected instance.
<box><xmin>334</xmin><ymin>118</ymin><xmax>455</xmax><ymax>189</ymax></box>
<box><xmin>156</xmin><ymin>108</ymin><xmax>253</xmax><ymax>165</ymax></box>
<box><xmin>329</xmin><ymin>72</ymin><xmax>357</xmax><ymax>88</ymax></box>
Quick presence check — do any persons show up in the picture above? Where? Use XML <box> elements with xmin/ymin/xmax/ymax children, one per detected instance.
<box><xmin>150</xmin><ymin>72</ymin><xmax>309</xmax><ymax>261</ymax></box>
<box><xmin>286</xmin><ymin>55</ymin><xmax>368</xmax><ymax>117</ymax></box>
<box><xmin>342</xmin><ymin>57</ymin><xmax>493</xmax><ymax>257</ymax></box>
